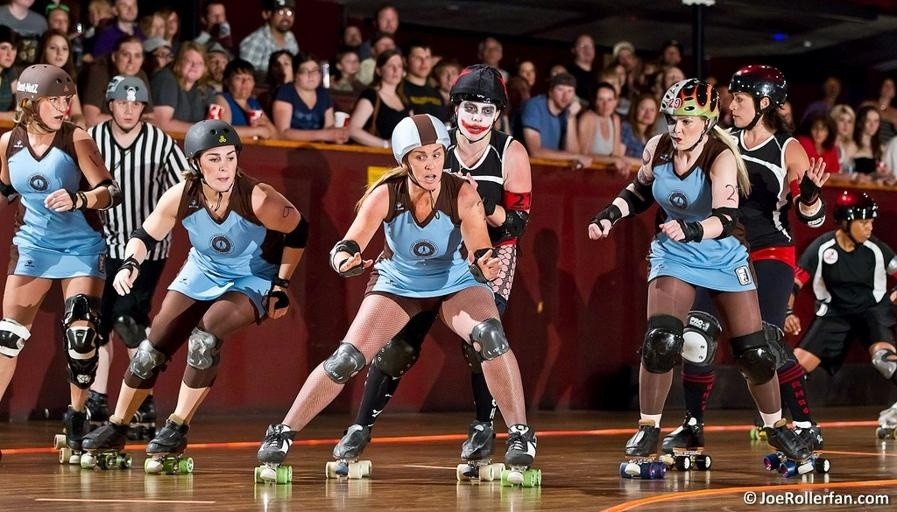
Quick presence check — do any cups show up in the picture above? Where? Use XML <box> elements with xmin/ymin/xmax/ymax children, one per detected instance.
<box><xmin>207</xmin><ymin>103</ymin><xmax>225</xmax><ymax>119</ymax></box>
<box><xmin>63</xmin><ymin>94</ymin><xmax>76</xmax><ymax>121</ymax></box>
<box><xmin>877</xmin><ymin>162</ymin><xmax>888</xmax><ymax>171</ymax></box>
<box><xmin>334</xmin><ymin>111</ymin><xmax>349</xmax><ymax>128</ymax></box>
<box><xmin>218</xmin><ymin>21</ymin><xmax>234</xmax><ymax>48</ymax></box>
<box><xmin>249</xmin><ymin>110</ymin><xmax>263</xmax><ymax>128</ymax></box>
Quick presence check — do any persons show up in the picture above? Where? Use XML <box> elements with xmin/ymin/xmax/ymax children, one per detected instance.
<box><xmin>1</xmin><ymin>63</ymin><xmax>124</xmax><ymax>451</ymax></box>
<box><xmin>588</xmin><ymin>77</ymin><xmax>813</xmax><ymax>464</ymax></box>
<box><xmin>778</xmin><ymin>189</ymin><xmax>897</xmax><ymax>436</ymax></box>
<box><xmin>256</xmin><ymin>113</ymin><xmax>537</xmax><ymax>465</ymax></box>
<box><xmin>333</xmin><ymin>62</ymin><xmax>533</xmax><ymax>460</ymax></box>
<box><xmin>660</xmin><ymin>63</ymin><xmax>831</xmax><ymax>451</ymax></box>
<box><xmin>82</xmin><ymin>118</ymin><xmax>310</xmax><ymax>454</ymax></box>
<box><xmin>84</xmin><ymin>73</ymin><xmax>191</xmax><ymax>423</ymax></box>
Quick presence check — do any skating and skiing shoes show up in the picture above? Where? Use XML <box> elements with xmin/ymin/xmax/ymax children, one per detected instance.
<box><xmin>662</xmin><ymin>412</ymin><xmax>714</xmax><ymax>472</ymax></box>
<box><xmin>324</xmin><ymin>424</ymin><xmax>375</xmax><ymax>481</ymax></box>
<box><xmin>80</xmin><ymin>417</ymin><xmax>133</xmax><ymax>472</ymax></box>
<box><xmin>145</xmin><ymin>415</ymin><xmax>196</xmax><ymax>478</ymax></box>
<box><xmin>750</xmin><ymin>411</ymin><xmax>831</xmax><ymax>478</ymax></box>
<box><xmin>457</xmin><ymin>418</ymin><xmax>506</xmax><ymax>485</ymax></box>
<box><xmin>619</xmin><ymin>418</ymin><xmax>667</xmax><ymax>481</ymax></box>
<box><xmin>501</xmin><ymin>424</ymin><xmax>542</xmax><ymax>489</ymax></box>
<box><xmin>251</xmin><ymin>423</ymin><xmax>297</xmax><ymax>486</ymax></box>
<box><xmin>83</xmin><ymin>388</ymin><xmax>159</xmax><ymax>442</ymax></box>
<box><xmin>53</xmin><ymin>404</ymin><xmax>91</xmax><ymax>467</ymax></box>
<box><xmin>874</xmin><ymin>401</ymin><xmax>897</xmax><ymax>441</ymax></box>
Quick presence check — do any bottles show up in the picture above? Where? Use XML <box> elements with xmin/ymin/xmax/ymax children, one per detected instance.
<box><xmin>320</xmin><ymin>60</ymin><xmax>330</xmax><ymax>88</ymax></box>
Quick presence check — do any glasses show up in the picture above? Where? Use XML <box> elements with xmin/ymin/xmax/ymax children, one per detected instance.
<box><xmin>44</xmin><ymin>4</ymin><xmax>70</xmax><ymax>16</ymax></box>
<box><xmin>275</xmin><ymin>9</ymin><xmax>293</xmax><ymax>16</ymax></box>
<box><xmin>154</xmin><ymin>52</ymin><xmax>174</xmax><ymax>61</ymax></box>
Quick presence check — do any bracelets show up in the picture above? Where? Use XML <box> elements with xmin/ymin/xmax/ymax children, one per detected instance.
<box><xmin>332</xmin><ymin>237</ymin><xmax>361</xmax><ymax>254</ymax></box>
<box><xmin>272</xmin><ymin>276</ymin><xmax>290</xmax><ymax>289</ymax></box>
<box><xmin>481</xmin><ymin>195</ymin><xmax>497</xmax><ymax>217</ymax></box>
<box><xmin>77</xmin><ymin>188</ymin><xmax>88</xmax><ymax>212</ymax></box>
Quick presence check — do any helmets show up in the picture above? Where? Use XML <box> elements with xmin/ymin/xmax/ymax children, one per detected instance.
<box><xmin>183</xmin><ymin>118</ymin><xmax>243</xmax><ymax>162</ymax></box>
<box><xmin>833</xmin><ymin>190</ymin><xmax>878</xmax><ymax>223</ymax></box>
<box><xmin>727</xmin><ymin>62</ymin><xmax>788</xmax><ymax>110</ymax></box>
<box><xmin>390</xmin><ymin>113</ymin><xmax>451</xmax><ymax>169</ymax></box>
<box><xmin>657</xmin><ymin>77</ymin><xmax>722</xmax><ymax>122</ymax></box>
<box><xmin>16</xmin><ymin>63</ymin><xmax>76</xmax><ymax>101</ymax></box>
<box><xmin>103</xmin><ymin>75</ymin><xmax>150</xmax><ymax>108</ymax></box>
<box><xmin>447</xmin><ymin>63</ymin><xmax>508</xmax><ymax>113</ymax></box>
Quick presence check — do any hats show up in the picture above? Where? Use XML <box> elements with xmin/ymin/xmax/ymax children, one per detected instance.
<box><xmin>274</xmin><ymin>0</ymin><xmax>297</xmax><ymax>10</ymax></box>
<box><xmin>142</xmin><ymin>37</ymin><xmax>169</xmax><ymax>51</ymax></box>
